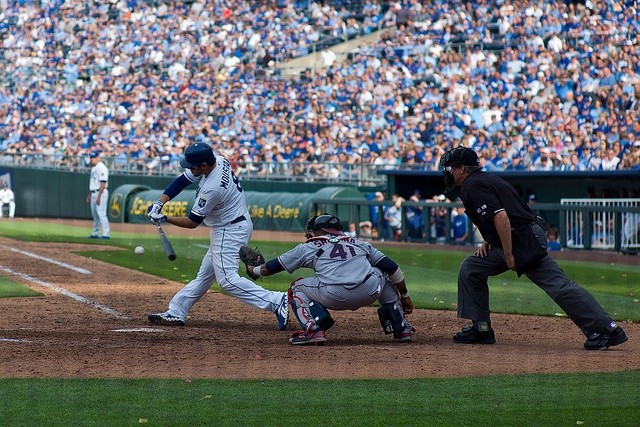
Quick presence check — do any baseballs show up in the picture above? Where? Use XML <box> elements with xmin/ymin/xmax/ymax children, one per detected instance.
<box><xmin>134</xmin><ymin>246</ymin><xmax>144</xmax><ymax>257</ymax></box>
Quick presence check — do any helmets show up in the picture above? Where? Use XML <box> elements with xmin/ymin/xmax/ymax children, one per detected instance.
<box><xmin>441</xmin><ymin>146</ymin><xmax>480</xmax><ymax>194</ymax></box>
<box><xmin>305</xmin><ymin>214</ymin><xmax>350</xmax><ymax>239</ymax></box>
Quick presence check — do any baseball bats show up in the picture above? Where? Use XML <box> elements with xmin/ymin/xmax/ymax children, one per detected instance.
<box><xmin>155</xmin><ymin>219</ymin><xmax>176</xmax><ymax>261</ymax></box>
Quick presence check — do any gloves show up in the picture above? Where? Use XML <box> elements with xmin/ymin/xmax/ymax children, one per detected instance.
<box><xmin>150</xmin><ymin>213</ymin><xmax>168</xmax><ymax>225</ymax></box>
<box><xmin>147</xmin><ymin>199</ymin><xmax>164</xmax><ymax>219</ymax></box>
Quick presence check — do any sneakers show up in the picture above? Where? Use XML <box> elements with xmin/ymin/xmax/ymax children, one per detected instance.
<box><xmin>452</xmin><ymin>325</ymin><xmax>497</xmax><ymax>345</ymax></box>
<box><xmin>583</xmin><ymin>325</ymin><xmax>628</xmax><ymax>351</ymax></box>
<box><xmin>87</xmin><ymin>234</ymin><xmax>99</xmax><ymax>238</ymax></box>
<box><xmin>394</xmin><ymin>322</ymin><xmax>413</xmax><ymax>342</ymax></box>
<box><xmin>101</xmin><ymin>235</ymin><xmax>110</xmax><ymax>240</ymax></box>
<box><xmin>274</xmin><ymin>292</ymin><xmax>289</xmax><ymax>330</ymax></box>
<box><xmin>292</xmin><ymin>328</ymin><xmax>328</xmax><ymax>345</ymax></box>
<box><xmin>148</xmin><ymin>311</ymin><xmax>186</xmax><ymax>325</ymax></box>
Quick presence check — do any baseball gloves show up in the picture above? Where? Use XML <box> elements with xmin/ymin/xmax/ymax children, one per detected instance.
<box><xmin>239</xmin><ymin>246</ymin><xmax>266</xmax><ymax>281</ymax></box>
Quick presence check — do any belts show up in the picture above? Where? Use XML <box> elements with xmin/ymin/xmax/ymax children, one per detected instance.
<box><xmin>231</xmin><ymin>216</ymin><xmax>246</xmax><ymax>224</ymax></box>
<box><xmin>92</xmin><ymin>189</ymin><xmax>99</xmax><ymax>193</ymax></box>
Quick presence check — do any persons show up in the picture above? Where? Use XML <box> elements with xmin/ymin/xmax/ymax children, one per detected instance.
<box><xmin>358</xmin><ymin>220</ymin><xmax>372</xmax><ymax>237</ymax></box>
<box><xmin>445</xmin><ymin>1</ymin><xmax>640</xmax><ymax>170</ymax></box>
<box><xmin>147</xmin><ymin>143</ymin><xmax>289</xmax><ymax>331</ymax></box>
<box><xmin>391</xmin><ymin>195</ymin><xmax>398</xmax><ymax>201</ymax></box>
<box><xmin>452</xmin><ymin>205</ymin><xmax>469</xmax><ymax>242</ymax></box>
<box><xmin>605</xmin><ymin>218</ymin><xmax>615</xmax><ymax>245</ymax></box>
<box><xmin>0</xmin><ymin>0</ymin><xmax>147</xmax><ymax>171</ymax></box>
<box><xmin>343</xmin><ymin>223</ymin><xmax>357</xmax><ymax>238</ymax></box>
<box><xmin>86</xmin><ymin>152</ymin><xmax>111</xmax><ymax>238</ymax></box>
<box><xmin>440</xmin><ymin>146</ymin><xmax>628</xmax><ymax>349</ymax></box>
<box><xmin>0</xmin><ymin>183</ymin><xmax>17</xmax><ymax>217</ymax></box>
<box><xmin>568</xmin><ymin>211</ymin><xmax>583</xmax><ymax>246</ymax></box>
<box><xmin>244</xmin><ymin>213</ymin><xmax>414</xmax><ymax>344</ymax></box>
<box><xmin>370</xmin><ymin>228</ymin><xmax>380</xmax><ymax>241</ymax></box>
<box><xmin>430</xmin><ymin>195</ymin><xmax>452</xmax><ymax>240</ymax></box>
<box><xmin>529</xmin><ymin>194</ymin><xmax>536</xmax><ymax>205</ymax></box>
<box><xmin>545</xmin><ymin>227</ymin><xmax>561</xmax><ymax>251</ymax></box>
<box><xmin>295</xmin><ymin>0</ymin><xmax>446</xmax><ymax>174</ymax></box>
<box><xmin>364</xmin><ymin>191</ymin><xmax>385</xmax><ymax>227</ymax></box>
<box><xmin>592</xmin><ymin>220</ymin><xmax>608</xmax><ymax>244</ymax></box>
<box><xmin>384</xmin><ymin>197</ymin><xmax>404</xmax><ymax>240</ymax></box>
<box><xmin>405</xmin><ymin>190</ymin><xmax>422</xmax><ymax>241</ymax></box>
<box><xmin>147</xmin><ymin>0</ymin><xmax>295</xmax><ymax>176</ymax></box>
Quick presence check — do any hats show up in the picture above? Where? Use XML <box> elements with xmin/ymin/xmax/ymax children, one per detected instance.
<box><xmin>90</xmin><ymin>150</ymin><xmax>98</xmax><ymax>158</ymax></box>
<box><xmin>180</xmin><ymin>143</ymin><xmax>213</xmax><ymax>169</ymax></box>
